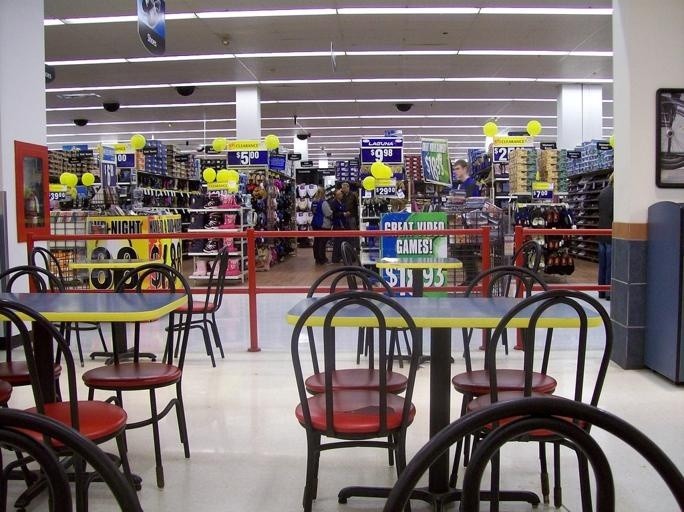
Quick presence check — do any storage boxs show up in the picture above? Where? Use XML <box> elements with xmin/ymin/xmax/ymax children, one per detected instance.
<box><xmin>466</xmin><ymin>148</ymin><xmax>493</xmax><ymax>179</ymax></box>
<box><xmin>134</xmin><ymin>140</ymin><xmax>201</xmax><ymax>179</ymax></box>
<box><xmin>508</xmin><ymin>138</ymin><xmax>613</xmax><ymax>192</ymax></box>
<box><xmin>332</xmin><ymin>161</ymin><xmax>359</xmax><ymax>183</ymax></box>
<box><xmin>400</xmin><ymin>153</ymin><xmax>424</xmax><ymax>183</ymax></box>
<box><xmin>47</xmin><ymin>149</ymin><xmax>101</xmax><ymax>184</ymax></box>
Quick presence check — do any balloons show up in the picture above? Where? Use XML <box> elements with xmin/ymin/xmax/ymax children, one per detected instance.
<box><xmin>483</xmin><ymin>122</ymin><xmax>497</xmax><ymax>137</ymax></box>
<box><xmin>216</xmin><ymin>169</ymin><xmax>239</xmax><ymax>183</ymax></box>
<box><xmin>527</xmin><ymin>121</ymin><xmax>542</xmax><ymax>136</ymax></box>
<box><xmin>213</xmin><ymin>137</ymin><xmax>227</xmax><ymax>151</ymax></box>
<box><xmin>610</xmin><ymin>135</ymin><xmax>615</xmax><ymax>149</ymax></box>
<box><xmin>371</xmin><ymin>162</ymin><xmax>393</xmax><ymax>180</ymax></box>
<box><xmin>131</xmin><ymin>135</ymin><xmax>146</xmax><ymax>150</ymax></box>
<box><xmin>82</xmin><ymin>173</ymin><xmax>95</xmax><ymax>187</ymax></box>
<box><xmin>60</xmin><ymin>172</ymin><xmax>78</xmax><ymax>187</ymax></box>
<box><xmin>363</xmin><ymin>177</ymin><xmax>375</xmax><ymax>190</ymax></box>
<box><xmin>203</xmin><ymin>168</ymin><xmax>216</xmax><ymax>183</ymax></box>
<box><xmin>265</xmin><ymin>135</ymin><xmax>279</xmax><ymax>150</ymax></box>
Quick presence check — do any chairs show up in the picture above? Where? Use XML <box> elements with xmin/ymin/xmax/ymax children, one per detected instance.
<box><xmin>1</xmin><ymin>298</ymin><xmax>137</xmax><ymax>512</ymax></box>
<box><xmin>291</xmin><ymin>291</ymin><xmax>420</xmax><ymax>511</ymax></box>
<box><xmin>83</xmin><ymin>263</ymin><xmax>192</xmax><ymax>489</ymax></box>
<box><xmin>29</xmin><ymin>247</ymin><xmax>107</xmax><ymax>366</ymax></box>
<box><xmin>383</xmin><ymin>398</ymin><xmax>684</xmax><ymax>512</ymax></box>
<box><xmin>306</xmin><ymin>266</ymin><xmax>410</xmax><ymax>466</ymax></box>
<box><xmin>0</xmin><ymin>266</ymin><xmax>66</xmax><ymax>404</ymax></box>
<box><xmin>1</xmin><ymin>408</ymin><xmax>143</xmax><ymax>512</ymax></box>
<box><xmin>451</xmin><ymin>288</ymin><xmax>614</xmax><ymax>512</ymax></box>
<box><xmin>163</xmin><ymin>246</ymin><xmax>230</xmax><ymax>367</ymax></box>
<box><xmin>452</xmin><ymin>266</ymin><xmax>558</xmax><ymax>468</ymax></box>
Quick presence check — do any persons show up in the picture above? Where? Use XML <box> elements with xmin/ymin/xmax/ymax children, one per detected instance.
<box><xmin>311</xmin><ymin>189</ymin><xmax>334</xmax><ymax>264</ymax></box>
<box><xmin>444</xmin><ymin>159</ymin><xmax>469</xmax><ymax>197</ymax></box>
<box><xmin>456</xmin><ymin>160</ymin><xmax>481</xmax><ymax>197</ymax></box>
<box><xmin>341</xmin><ymin>182</ymin><xmax>358</xmax><ymax>262</ymax></box>
<box><xmin>597</xmin><ymin>171</ymin><xmax>613</xmax><ymax>301</ymax></box>
<box><xmin>327</xmin><ymin>186</ymin><xmax>351</xmax><ymax>265</ymax></box>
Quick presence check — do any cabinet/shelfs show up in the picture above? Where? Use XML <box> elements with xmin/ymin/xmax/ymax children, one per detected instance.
<box><xmin>116</xmin><ymin>169</ymin><xmax>207</xmax><ymax>260</ymax></box>
<box><xmin>377</xmin><ymin>212</ymin><xmax>464</xmax><ymax>300</ymax></box>
<box><xmin>187</xmin><ymin>206</ymin><xmax>255</xmax><ymax>284</ymax></box>
<box><xmin>359</xmin><ymin>189</ymin><xmax>505</xmax><ymax>265</ymax></box>
<box><xmin>228</xmin><ymin>166</ymin><xmax>297</xmax><ymax>270</ymax></box>
<box><xmin>86</xmin><ymin>215</ymin><xmax>182</xmax><ymax>291</ymax></box>
<box><xmin>560</xmin><ymin>169</ymin><xmax>614</xmax><ymax>263</ymax></box>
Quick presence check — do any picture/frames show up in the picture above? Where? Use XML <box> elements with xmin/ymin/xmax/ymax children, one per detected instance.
<box><xmin>655</xmin><ymin>88</ymin><xmax>684</xmax><ymax>189</ymax></box>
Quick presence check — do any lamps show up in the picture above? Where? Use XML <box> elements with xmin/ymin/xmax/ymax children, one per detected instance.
<box><xmin>44</xmin><ymin>8</ymin><xmax>615</xmax><ymax>24</ymax></box>
<box><xmin>46</xmin><ymin>75</ymin><xmax>610</xmax><ymax>92</ymax></box>
<box><xmin>45</xmin><ymin>51</ymin><xmax>613</xmax><ymax>67</ymax></box>
<box><xmin>52</xmin><ymin>115</ymin><xmax>614</xmax><ymax>161</ymax></box>
<box><xmin>46</xmin><ymin>97</ymin><xmax>614</xmax><ymax>112</ymax></box>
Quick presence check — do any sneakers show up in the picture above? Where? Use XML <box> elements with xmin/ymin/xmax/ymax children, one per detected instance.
<box><xmin>547</xmin><ymin>237</ymin><xmax>553</xmax><ymax>253</ymax></box>
<box><xmin>568</xmin><ymin>256</ymin><xmax>574</xmax><ymax>275</ymax></box>
<box><xmin>554</xmin><ymin>238</ymin><xmax>560</xmax><ymax>252</ymax></box>
<box><xmin>529</xmin><ymin>209</ymin><xmax>539</xmax><ymax>228</ymax></box>
<box><xmin>535</xmin><ymin>206</ymin><xmax>546</xmax><ymax>228</ymax></box>
<box><xmin>561</xmin><ymin>256</ymin><xmax>567</xmax><ymax>275</ymax></box>
<box><xmin>554</xmin><ymin>256</ymin><xmax>561</xmax><ymax>273</ymax></box>
<box><xmin>547</xmin><ymin>256</ymin><xmax>553</xmax><ymax>273</ymax></box>
<box><xmin>515</xmin><ymin>207</ymin><xmax>529</xmax><ymax>226</ymax></box>
<box><xmin>547</xmin><ymin>207</ymin><xmax>575</xmax><ymax>229</ymax></box>
